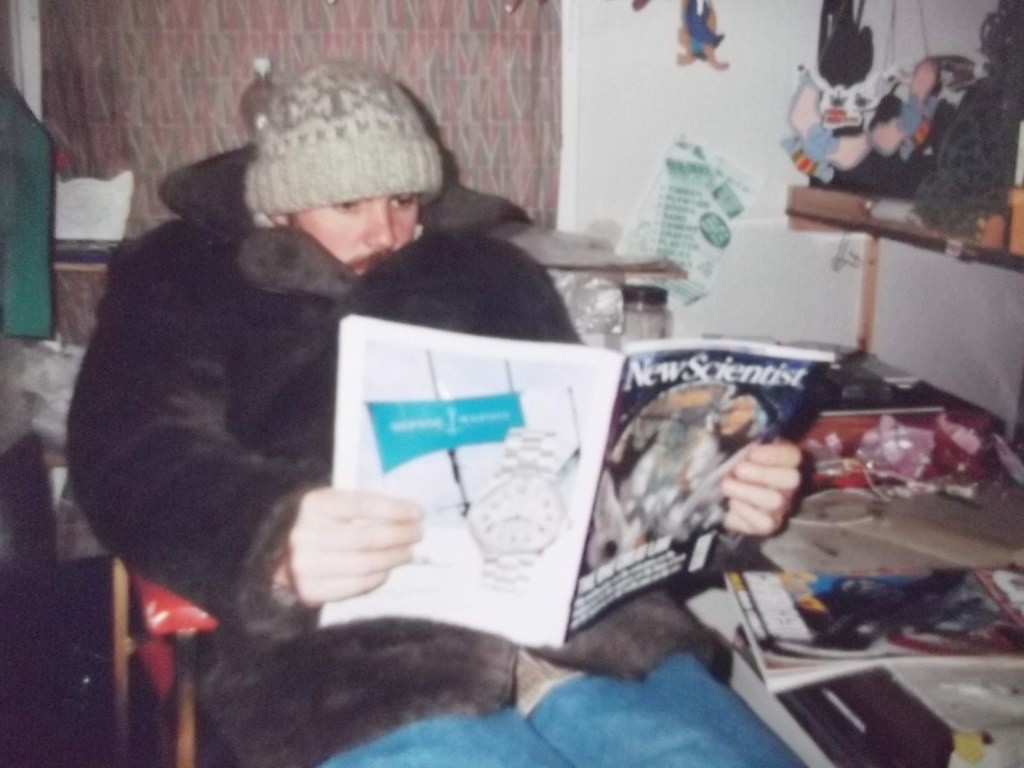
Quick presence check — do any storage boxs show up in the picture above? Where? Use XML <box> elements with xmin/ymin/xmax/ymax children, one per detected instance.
<box><xmin>794</xmin><ymin>373</ymin><xmax>1003</xmax><ymax>493</ymax></box>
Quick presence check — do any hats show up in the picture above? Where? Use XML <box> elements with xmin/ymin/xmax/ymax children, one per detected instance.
<box><xmin>258</xmin><ymin>61</ymin><xmax>444</xmax><ymax>215</ymax></box>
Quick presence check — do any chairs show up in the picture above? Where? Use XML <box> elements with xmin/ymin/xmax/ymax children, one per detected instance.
<box><xmin>109</xmin><ymin>553</ymin><xmax>219</xmax><ymax>768</ymax></box>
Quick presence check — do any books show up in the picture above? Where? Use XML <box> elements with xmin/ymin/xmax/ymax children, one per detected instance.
<box><xmin>723</xmin><ymin>564</ymin><xmax>1024</xmax><ymax>694</ymax></box>
<box><xmin>318</xmin><ymin>313</ymin><xmax>834</xmax><ymax>648</ymax></box>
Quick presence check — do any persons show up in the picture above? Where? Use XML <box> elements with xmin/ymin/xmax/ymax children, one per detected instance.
<box><xmin>652</xmin><ymin>395</ymin><xmax>758</xmax><ymax>549</ymax></box>
<box><xmin>812</xmin><ymin>566</ymin><xmax>983</xmax><ymax>648</ymax></box>
<box><xmin>66</xmin><ymin>65</ymin><xmax>805</xmax><ymax>768</ymax></box>
